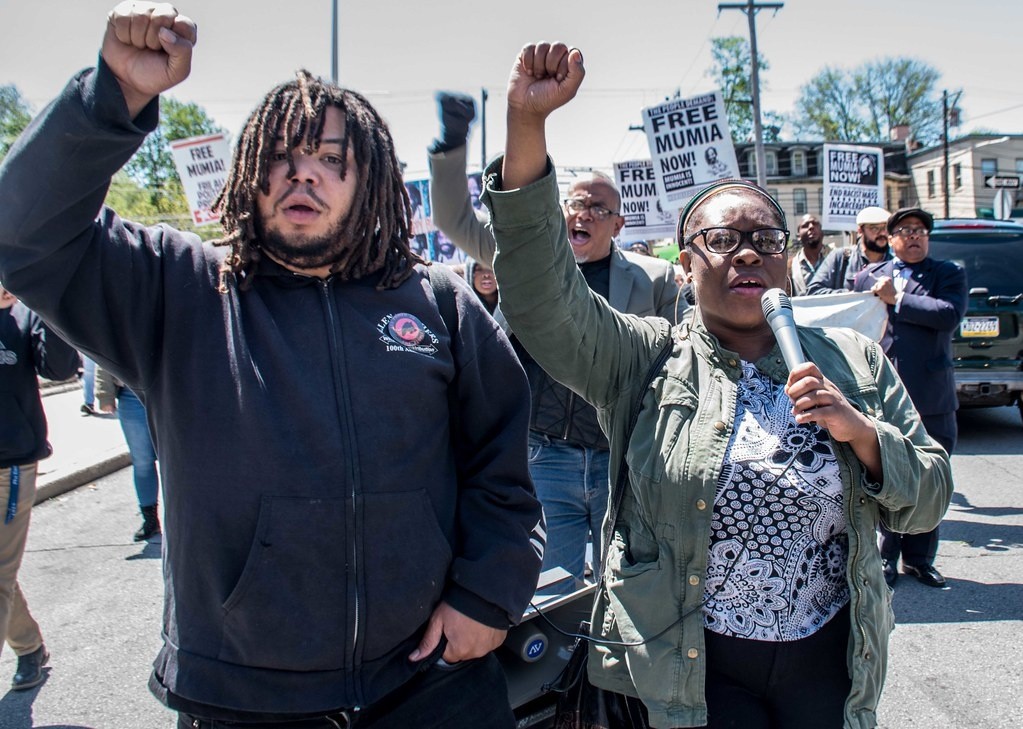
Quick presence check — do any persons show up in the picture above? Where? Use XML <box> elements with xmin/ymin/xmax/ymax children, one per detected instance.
<box><xmin>0</xmin><ymin>0</ymin><xmax>545</xmax><ymax>729</ymax></box>
<box><xmin>0</xmin><ymin>281</ymin><xmax>80</xmax><ymax>690</ymax></box>
<box><xmin>673</xmin><ymin>267</ymin><xmax>686</xmax><ymax>288</ymax></box>
<box><xmin>853</xmin><ymin>208</ymin><xmax>968</xmax><ymax>587</ymax></box>
<box><xmin>94</xmin><ymin>360</ymin><xmax>165</xmax><ymax>541</ymax></box>
<box><xmin>628</xmin><ymin>242</ymin><xmax>651</xmax><ymax>254</ymax></box>
<box><xmin>81</xmin><ymin>353</ymin><xmax>95</xmax><ymax>414</ymax></box>
<box><xmin>478</xmin><ymin>41</ymin><xmax>954</xmax><ymax>729</ymax></box>
<box><xmin>425</xmin><ymin>85</ymin><xmax>689</xmax><ymax>596</ymax></box>
<box><xmin>787</xmin><ymin>213</ymin><xmax>836</xmax><ymax>297</ymax></box>
<box><xmin>805</xmin><ymin>207</ymin><xmax>893</xmax><ymax>293</ymax></box>
<box><xmin>463</xmin><ymin>255</ymin><xmax>499</xmax><ymax>315</ymax></box>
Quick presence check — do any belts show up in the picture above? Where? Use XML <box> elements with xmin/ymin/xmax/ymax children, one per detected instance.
<box><xmin>180</xmin><ymin>673</ymin><xmax>418</xmax><ymax>729</ymax></box>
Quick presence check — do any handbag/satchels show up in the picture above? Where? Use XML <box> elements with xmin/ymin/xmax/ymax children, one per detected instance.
<box><xmin>539</xmin><ymin>620</ymin><xmax>651</xmax><ymax>729</ymax></box>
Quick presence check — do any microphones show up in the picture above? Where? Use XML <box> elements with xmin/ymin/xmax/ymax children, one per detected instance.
<box><xmin>761</xmin><ymin>288</ymin><xmax>818</xmax><ymax>426</ymax></box>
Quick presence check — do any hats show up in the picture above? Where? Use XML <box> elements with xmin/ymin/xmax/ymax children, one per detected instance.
<box><xmin>888</xmin><ymin>205</ymin><xmax>934</xmax><ymax>239</ymax></box>
<box><xmin>857</xmin><ymin>206</ymin><xmax>893</xmax><ymax>225</ymax></box>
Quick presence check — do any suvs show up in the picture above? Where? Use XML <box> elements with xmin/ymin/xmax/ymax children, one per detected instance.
<box><xmin>855</xmin><ymin>217</ymin><xmax>1023</xmax><ymax>422</ymax></box>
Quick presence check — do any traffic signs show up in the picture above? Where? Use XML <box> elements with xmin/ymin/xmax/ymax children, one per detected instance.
<box><xmin>984</xmin><ymin>175</ymin><xmax>1020</xmax><ymax>190</ymax></box>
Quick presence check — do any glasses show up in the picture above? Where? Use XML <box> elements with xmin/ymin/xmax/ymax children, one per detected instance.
<box><xmin>563</xmin><ymin>197</ymin><xmax>621</xmax><ymax>221</ymax></box>
<box><xmin>684</xmin><ymin>226</ymin><xmax>790</xmax><ymax>256</ymax></box>
<box><xmin>863</xmin><ymin>226</ymin><xmax>888</xmax><ymax>235</ymax></box>
<box><xmin>892</xmin><ymin>224</ymin><xmax>930</xmax><ymax>236</ymax></box>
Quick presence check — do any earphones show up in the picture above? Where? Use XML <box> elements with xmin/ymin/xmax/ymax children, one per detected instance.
<box><xmin>688</xmin><ymin>271</ymin><xmax>692</xmax><ymax>279</ymax></box>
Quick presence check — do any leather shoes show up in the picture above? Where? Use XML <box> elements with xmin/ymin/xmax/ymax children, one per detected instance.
<box><xmin>882</xmin><ymin>557</ymin><xmax>899</xmax><ymax>587</ymax></box>
<box><xmin>901</xmin><ymin>557</ymin><xmax>946</xmax><ymax>587</ymax></box>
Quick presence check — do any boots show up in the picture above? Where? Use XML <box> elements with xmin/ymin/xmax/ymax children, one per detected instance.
<box><xmin>134</xmin><ymin>503</ymin><xmax>161</xmax><ymax>542</ymax></box>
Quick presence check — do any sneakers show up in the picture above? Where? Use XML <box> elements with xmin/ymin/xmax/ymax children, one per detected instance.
<box><xmin>81</xmin><ymin>403</ymin><xmax>95</xmax><ymax>415</ymax></box>
<box><xmin>11</xmin><ymin>646</ymin><xmax>49</xmax><ymax>688</ymax></box>
